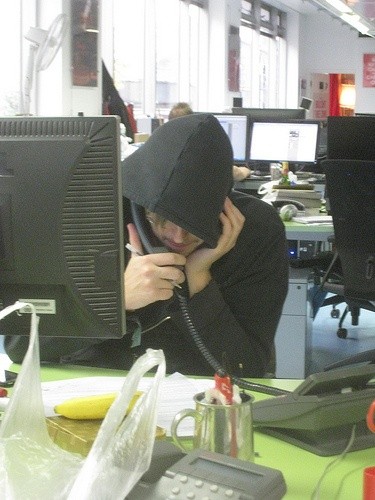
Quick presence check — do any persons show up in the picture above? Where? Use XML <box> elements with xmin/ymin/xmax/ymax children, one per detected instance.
<box><xmin>4</xmin><ymin>113</ymin><xmax>289</xmax><ymax>378</ymax></box>
<box><xmin>169</xmin><ymin>102</ymin><xmax>253</xmax><ymax>182</ymax></box>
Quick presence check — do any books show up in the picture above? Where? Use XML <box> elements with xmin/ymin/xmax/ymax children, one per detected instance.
<box><xmin>293</xmin><ymin>215</ymin><xmax>334</xmax><ymax>226</ymax></box>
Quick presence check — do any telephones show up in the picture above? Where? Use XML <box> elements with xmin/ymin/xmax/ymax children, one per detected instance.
<box><xmin>122</xmin><ymin>200</ymin><xmax>184</xmax><ymax>271</ymax></box>
<box><xmin>124</xmin><ymin>436</ymin><xmax>286</xmax><ymax>500</ymax></box>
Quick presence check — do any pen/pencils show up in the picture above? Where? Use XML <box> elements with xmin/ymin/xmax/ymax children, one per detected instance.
<box><xmin>215</xmin><ymin>368</ymin><xmax>232</xmax><ymax>405</ymax></box>
<box><xmin>124</xmin><ymin>240</ymin><xmax>182</xmax><ymax>289</ymax></box>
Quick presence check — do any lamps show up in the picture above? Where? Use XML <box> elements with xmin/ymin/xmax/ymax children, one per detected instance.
<box><xmin>340</xmin><ymin>84</ymin><xmax>356</xmax><ymax>109</ymax></box>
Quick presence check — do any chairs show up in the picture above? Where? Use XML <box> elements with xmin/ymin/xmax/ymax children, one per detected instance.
<box><xmin>307</xmin><ymin>158</ymin><xmax>375</xmax><ymax>340</ymax></box>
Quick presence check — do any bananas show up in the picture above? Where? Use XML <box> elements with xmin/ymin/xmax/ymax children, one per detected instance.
<box><xmin>54</xmin><ymin>390</ymin><xmax>145</xmax><ymax>420</ymax></box>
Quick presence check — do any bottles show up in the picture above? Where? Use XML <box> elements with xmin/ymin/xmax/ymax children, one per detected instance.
<box><xmin>282</xmin><ymin>162</ymin><xmax>289</xmax><ymax>180</ymax></box>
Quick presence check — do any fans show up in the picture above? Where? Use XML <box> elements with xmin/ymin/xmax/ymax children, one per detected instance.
<box><xmin>18</xmin><ymin>12</ymin><xmax>69</xmax><ymax>116</ymax></box>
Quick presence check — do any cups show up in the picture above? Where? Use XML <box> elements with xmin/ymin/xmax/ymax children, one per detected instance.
<box><xmin>270</xmin><ymin>162</ymin><xmax>282</xmax><ymax>181</ymax></box>
<box><xmin>169</xmin><ymin>391</ymin><xmax>255</xmax><ymax>465</ymax></box>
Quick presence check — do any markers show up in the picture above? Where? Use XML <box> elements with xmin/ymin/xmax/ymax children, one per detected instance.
<box><xmin>0</xmin><ymin>387</ymin><xmax>7</xmax><ymax>396</ymax></box>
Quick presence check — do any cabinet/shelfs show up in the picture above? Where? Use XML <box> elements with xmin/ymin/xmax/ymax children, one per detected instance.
<box><xmin>270</xmin><ymin>267</ymin><xmax>311</xmax><ymax>381</ymax></box>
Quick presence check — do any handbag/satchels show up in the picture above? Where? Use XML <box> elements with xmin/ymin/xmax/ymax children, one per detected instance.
<box><xmin>0</xmin><ymin>302</ymin><xmax>166</xmax><ymax>500</ymax></box>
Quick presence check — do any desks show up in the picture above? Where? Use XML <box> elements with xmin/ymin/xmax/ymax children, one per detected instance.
<box><xmin>0</xmin><ymin>357</ymin><xmax>375</xmax><ymax>500</ymax></box>
<box><xmin>284</xmin><ymin>221</ymin><xmax>334</xmax><ymax>261</ymax></box>
<box><xmin>235</xmin><ymin>180</ymin><xmax>324</xmax><ymax>199</ymax></box>
